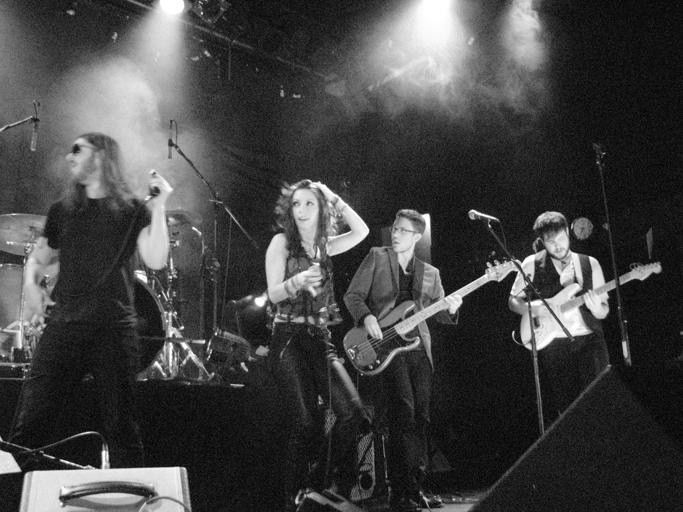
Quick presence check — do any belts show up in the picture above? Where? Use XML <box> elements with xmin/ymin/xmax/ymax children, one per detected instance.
<box><xmin>274</xmin><ymin>314</ymin><xmax>324</xmax><ymax>325</ymax></box>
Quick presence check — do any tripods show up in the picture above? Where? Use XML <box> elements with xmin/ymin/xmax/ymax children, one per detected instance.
<box><xmin>151</xmin><ymin>246</ymin><xmax>215</xmax><ymax>382</ymax></box>
<box><xmin>0</xmin><ymin>255</ymin><xmax>42</xmax><ymax>351</ymax></box>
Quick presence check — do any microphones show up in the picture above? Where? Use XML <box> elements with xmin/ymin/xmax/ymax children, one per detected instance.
<box><xmin>167</xmin><ymin>120</ymin><xmax>173</xmax><ymax>160</ymax></box>
<box><xmin>468</xmin><ymin>209</ymin><xmax>499</xmax><ymax>223</ymax></box>
<box><xmin>297</xmin><ymin>265</ymin><xmax>321</xmax><ymax>296</ymax></box>
<box><xmin>30</xmin><ymin>115</ymin><xmax>38</xmax><ymax>152</ymax></box>
<box><xmin>148</xmin><ymin>169</ymin><xmax>159</xmax><ymax>196</ymax></box>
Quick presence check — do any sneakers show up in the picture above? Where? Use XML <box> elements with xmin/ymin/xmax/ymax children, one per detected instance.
<box><xmin>390</xmin><ymin>491</ymin><xmax>442</xmax><ymax>512</ymax></box>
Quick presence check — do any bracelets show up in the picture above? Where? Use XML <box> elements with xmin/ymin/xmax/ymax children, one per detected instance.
<box><xmin>331</xmin><ymin>193</ymin><xmax>341</xmax><ymax>206</ymax></box>
<box><xmin>282</xmin><ymin>275</ymin><xmax>298</xmax><ymax>299</ymax></box>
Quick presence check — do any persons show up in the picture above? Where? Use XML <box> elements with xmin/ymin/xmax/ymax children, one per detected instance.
<box><xmin>1</xmin><ymin>129</ymin><xmax>172</xmax><ymax>511</ymax></box>
<box><xmin>260</xmin><ymin>176</ymin><xmax>371</xmax><ymax>511</ymax></box>
<box><xmin>343</xmin><ymin>207</ymin><xmax>464</xmax><ymax>512</ymax></box>
<box><xmin>509</xmin><ymin>209</ymin><xmax>610</xmax><ymax>428</ymax></box>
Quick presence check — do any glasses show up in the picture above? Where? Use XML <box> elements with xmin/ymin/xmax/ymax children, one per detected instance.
<box><xmin>389</xmin><ymin>227</ymin><xmax>420</xmax><ymax>233</ymax></box>
<box><xmin>72</xmin><ymin>144</ymin><xmax>95</xmax><ymax>154</ymax></box>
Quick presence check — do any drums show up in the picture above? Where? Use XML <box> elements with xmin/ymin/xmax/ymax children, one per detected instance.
<box><xmin>25</xmin><ymin>278</ymin><xmax>164</xmax><ymax>377</ymax></box>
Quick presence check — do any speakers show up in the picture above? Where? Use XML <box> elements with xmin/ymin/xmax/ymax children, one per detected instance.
<box><xmin>296</xmin><ymin>489</ymin><xmax>370</xmax><ymax>512</ymax></box>
<box><xmin>467</xmin><ymin>361</ymin><xmax>683</xmax><ymax>512</ymax></box>
<box><xmin>17</xmin><ymin>466</ymin><xmax>193</xmax><ymax>512</ymax></box>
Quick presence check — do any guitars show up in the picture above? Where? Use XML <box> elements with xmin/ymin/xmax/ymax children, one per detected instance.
<box><xmin>519</xmin><ymin>261</ymin><xmax>662</xmax><ymax>351</ymax></box>
<box><xmin>342</xmin><ymin>258</ymin><xmax>521</xmax><ymax>375</ymax></box>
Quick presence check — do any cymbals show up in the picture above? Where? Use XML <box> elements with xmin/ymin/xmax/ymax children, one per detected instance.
<box><xmin>167</xmin><ymin>210</ymin><xmax>202</xmax><ymax>230</ymax></box>
<box><xmin>0</xmin><ymin>214</ymin><xmax>48</xmax><ymax>255</ymax></box>
